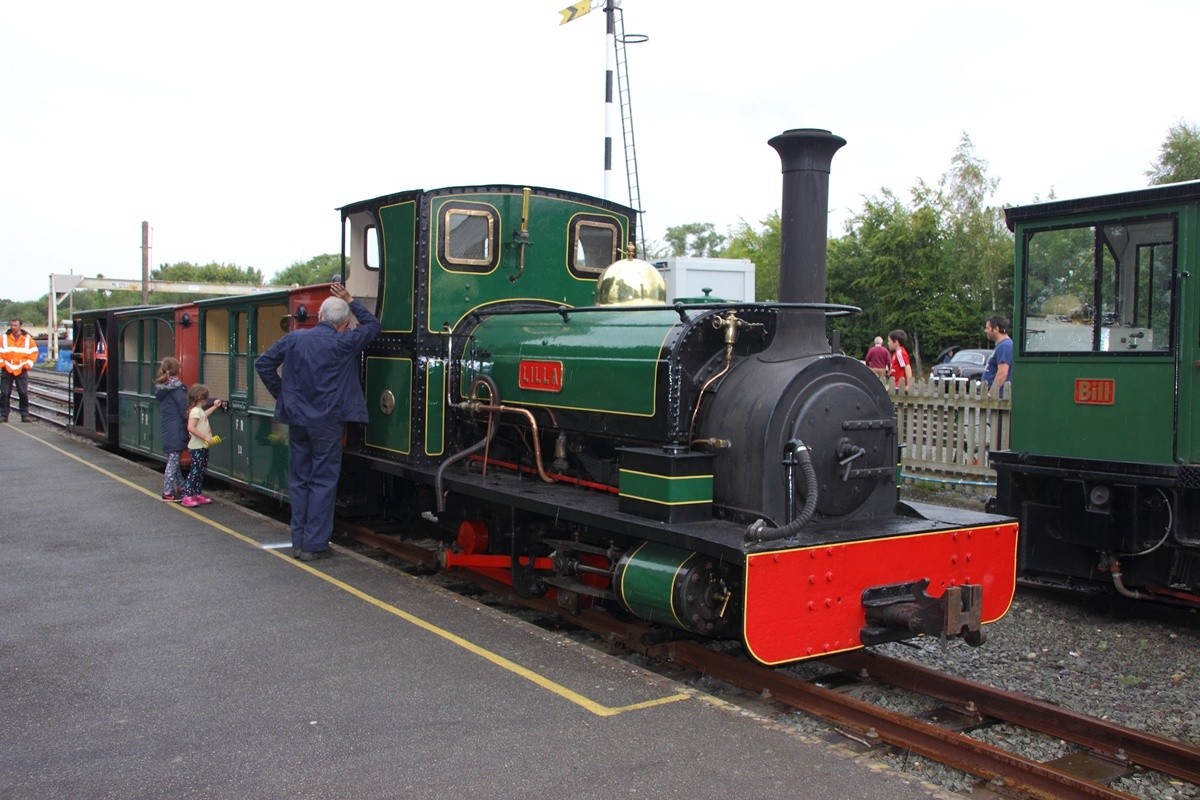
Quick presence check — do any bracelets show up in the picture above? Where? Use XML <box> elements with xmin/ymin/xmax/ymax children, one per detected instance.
<box><xmin>987</xmin><ymin>393</ymin><xmax>991</xmax><ymax>398</ymax></box>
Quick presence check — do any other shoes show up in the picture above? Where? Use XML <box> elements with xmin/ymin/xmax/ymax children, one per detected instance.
<box><xmin>294</xmin><ymin>548</ymin><xmax>301</xmax><ymax>557</ymax></box>
<box><xmin>22</xmin><ymin>416</ymin><xmax>32</xmax><ymax>422</ymax></box>
<box><xmin>0</xmin><ymin>415</ymin><xmax>8</xmax><ymax>422</ymax></box>
<box><xmin>300</xmin><ymin>546</ymin><xmax>332</xmax><ymax>561</ymax></box>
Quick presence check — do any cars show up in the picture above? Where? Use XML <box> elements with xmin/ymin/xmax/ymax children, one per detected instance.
<box><xmin>929</xmin><ymin>349</ymin><xmax>995</xmax><ymax>393</ymax></box>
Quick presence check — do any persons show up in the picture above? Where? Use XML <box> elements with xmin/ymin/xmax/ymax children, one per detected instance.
<box><xmin>253</xmin><ymin>280</ymin><xmax>382</xmax><ymax>561</ymax></box>
<box><xmin>888</xmin><ymin>329</ymin><xmax>913</xmax><ymax>396</ymax></box>
<box><xmin>153</xmin><ymin>358</ymin><xmax>190</xmax><ymax>503</ymax></box>
<box><xmin>980</xmin><ymin>316</ymin><xmax>1014</xmax><ymax>450</ymax></box>
<box><xmin>865</xmin><ymin>337</ymin><xmax>892</xmax><ymax>386</ymax></box>
<box><xmin>942</xmin><ymin>351</ymin><xmax>954</xmax><ymax>364</ymax></box>
<box><xmin>182</xmin><ymin>384</ymin><xmax>221</xmax><ymax>507</ymax></box>
<box><xmin>0</xmin><ymin>316</ymin><xmax>39</xmax><ymax>423</ymax></box>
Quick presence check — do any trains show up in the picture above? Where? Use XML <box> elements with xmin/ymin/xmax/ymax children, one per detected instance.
<box><xmin>68</xmin><ymin>126</ymin><xmax>1023</xmax><ymax>674</ymax></box>
<box><xmin>985</xmin><ymin>178</ymin><xmax>1199</xmax><ymax>600</ymax></box>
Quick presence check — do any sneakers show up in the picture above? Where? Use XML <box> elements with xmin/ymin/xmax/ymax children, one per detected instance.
<box><xmin>177</xmin><ymin>488</ymin><xmax>185</xmax><ymax>496</ymax></box>
<box><xmin>181</xmin><ymin>497</ymin><xmax>199</xmax><ymax>507</ymax></box>
<box><xmin>194</xmin><ymin>494</ymin><xmax>213</xmax><ymax>504</ymax></box>
<box><xmin>161</xmin><ymin>492</ymin><xmax>182</xmax><ymax>502</ymax></box>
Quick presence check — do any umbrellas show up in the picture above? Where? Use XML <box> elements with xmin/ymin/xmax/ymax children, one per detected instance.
<box><xmin>936</xmin><ymin>345</ymin><xmax>960</xmax><ymax>362</ymax></box>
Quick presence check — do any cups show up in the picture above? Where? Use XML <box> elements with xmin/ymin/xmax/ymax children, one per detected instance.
<box><xmin>203</xmin><ymin>434</ymin><xmax>222</xmax><ymax>449</ymax></box>
